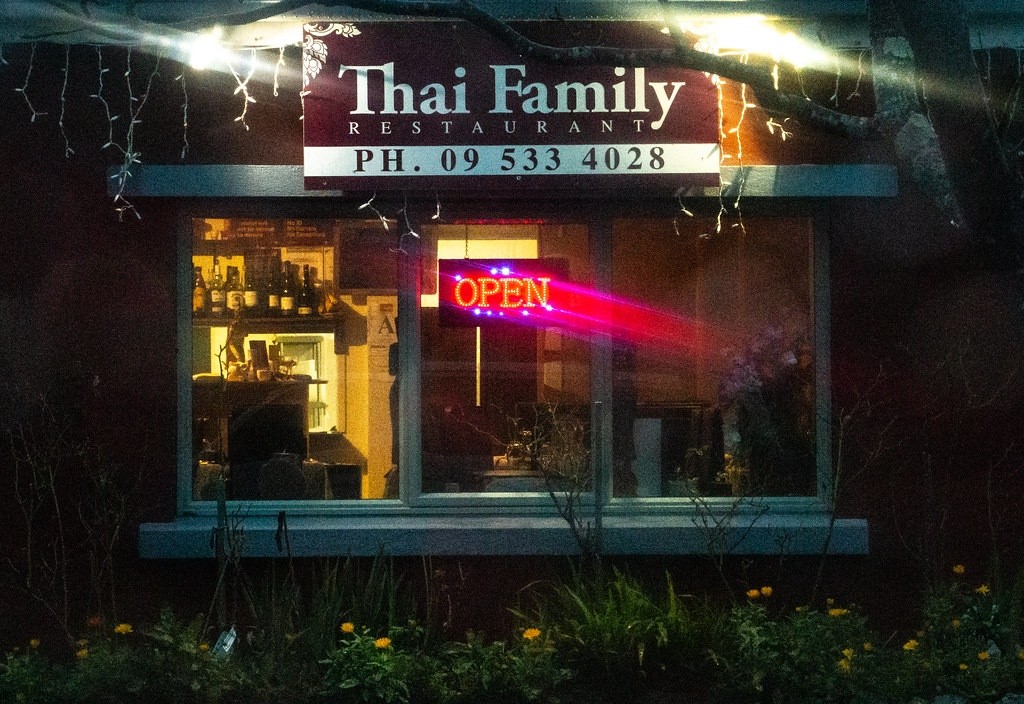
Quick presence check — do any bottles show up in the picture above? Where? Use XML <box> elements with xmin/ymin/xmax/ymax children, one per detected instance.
<box><xmin>297</xmin><ymin>265</ymin><xmax>316</xmax><ymax>316</ymax></box>
<box><xmin>192</xmin><ymin>267</ymin><xmax>207</xmax><ymax>319</ymax></box>
<box><xmin>208</xmin><ymin>265</ymin><xmax>225</xmax><ymax>319</ymax></box>
<box><xmin>226</xmin><ymin>260</ymin><xmax>294</xmax><ymax>318</ymax></box>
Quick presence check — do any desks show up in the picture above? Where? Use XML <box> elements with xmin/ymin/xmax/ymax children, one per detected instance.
<box><xmin>469</xmin><ymin>453</ymin><xmax>568</xmax><ymax>492</ymax></box>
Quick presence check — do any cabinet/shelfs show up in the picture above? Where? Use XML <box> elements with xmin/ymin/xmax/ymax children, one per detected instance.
<box><xmin>193</xmin><ymin>234</ymin><xmax>350</xmax><ymax>355</ymax></box>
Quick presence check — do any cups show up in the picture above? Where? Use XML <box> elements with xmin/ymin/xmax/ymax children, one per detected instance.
<box><xmin>257</xmin><ymin>370</ymin><xmax>272</xmax><ymax>382</ymax></box>
